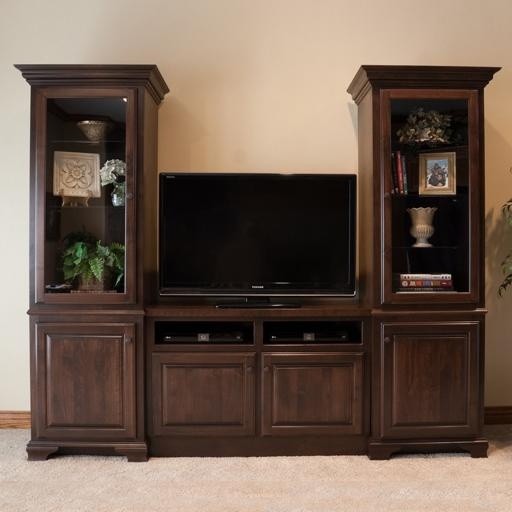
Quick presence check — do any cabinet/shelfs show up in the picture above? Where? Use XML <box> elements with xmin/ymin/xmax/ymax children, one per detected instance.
<box><xmin>346</xmin><ymin>65</ymin><xmax>503</xmax><ymax>460</ymax></box>
<box><xmin>14</xmin><ymin>64</ymin><xmax>170</xmax><ymax>463</ymax></box>
<box><xmin>145</xmin><ymin>300</ymin><xmax>372</xmax><ymax>456</ymax></box>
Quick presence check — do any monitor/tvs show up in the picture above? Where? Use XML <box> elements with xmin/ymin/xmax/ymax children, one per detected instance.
<box><xmin>156</xmin><ymin>171</ymin><xmax>357</xmax><ymax>310</ymax></box>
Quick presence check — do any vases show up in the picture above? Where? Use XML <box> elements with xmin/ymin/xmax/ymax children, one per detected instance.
<box><xmin>406</xmin><ymin>207</ymin><xmax>439</xmax><ymax>248</ymax></box>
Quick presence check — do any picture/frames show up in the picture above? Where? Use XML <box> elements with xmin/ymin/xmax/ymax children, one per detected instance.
<box><xmin>51</xmin><ymin>150</ymin><xmax>103</xmax><ymax>198</ymax></box>
<box><xmin>418</xmin><ymin>152</ymin><xmax>457</xmax><ymax>197</ymax></box>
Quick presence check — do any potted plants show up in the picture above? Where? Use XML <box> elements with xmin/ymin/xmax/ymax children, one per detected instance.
<box><xmin>59</xmin><ymin>235</ymin><xmax>125</xmax><ymax>292</ymax></box>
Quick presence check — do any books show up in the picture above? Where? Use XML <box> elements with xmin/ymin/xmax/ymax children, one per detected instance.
<box><xmin>395</xmin><ymin>269</ymin><xmax>456</xmax><ymax>292</ymax></box>
<box><xmin>392</xmin><ymin>150</ymin><xmax>409</xmax><ymax>194</ymax></box>
